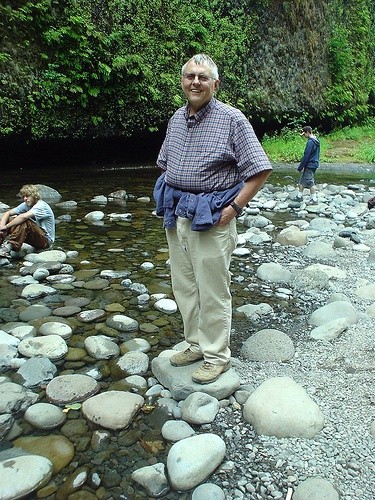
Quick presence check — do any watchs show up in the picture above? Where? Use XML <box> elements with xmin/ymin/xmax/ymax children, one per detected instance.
<box><xmin>230</xmin><ymin>202</ymin><xmax>245</xmax><ymax>219</ymax></box>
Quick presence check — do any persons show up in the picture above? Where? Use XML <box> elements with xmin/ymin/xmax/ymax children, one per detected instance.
<box><xmin>290</xmin><ymin>126</ymin><xmax>320</xmax><ymax>206</ymax></box>
<box><xmin>152</xmin><ymin>54</ymin><xmax>272</xmax><ymax>385</ymax></box>
<box><xmin>0</xmin><ymin>184</ymin><xmax>55</xmax><ymax>256</ymax></box>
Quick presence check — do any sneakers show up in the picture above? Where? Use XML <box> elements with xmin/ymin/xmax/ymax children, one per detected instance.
<box><xmin>306</xmin><ymin>198</ymin><xmax>318</xmax><ymax>205</ymax></box>
<box><xmin>290</xmin><ymin>195</ymin><xmax>303</xmax><ymax>201</ymax></box>
<box><xmin>192</xmin><ymin>361</ymin><xmax>232</xmax><ymax>385</ymax></box>
<box><xmin>170</xmin><ymin>349</ymin><xmax>203</xmax><ymax>367</ymax></box>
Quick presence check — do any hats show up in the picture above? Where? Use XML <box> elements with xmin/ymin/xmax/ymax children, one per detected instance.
<box><xmin>300</xmin><ymin>126</ymin><xmax>312</xmax><ymax>135</ymax></box>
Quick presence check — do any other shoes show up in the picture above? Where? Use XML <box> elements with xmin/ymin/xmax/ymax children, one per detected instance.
<box><xmin>0</xmin><ymin>242</ymin><xmax>12</xmax><ymax>258</ymax></box>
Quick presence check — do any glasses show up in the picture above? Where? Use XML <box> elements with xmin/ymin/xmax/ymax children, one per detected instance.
<box><xmin>184</xmin><ymin>75</ymin><xmax>217</xmax><ymax>82</ymax></box>
